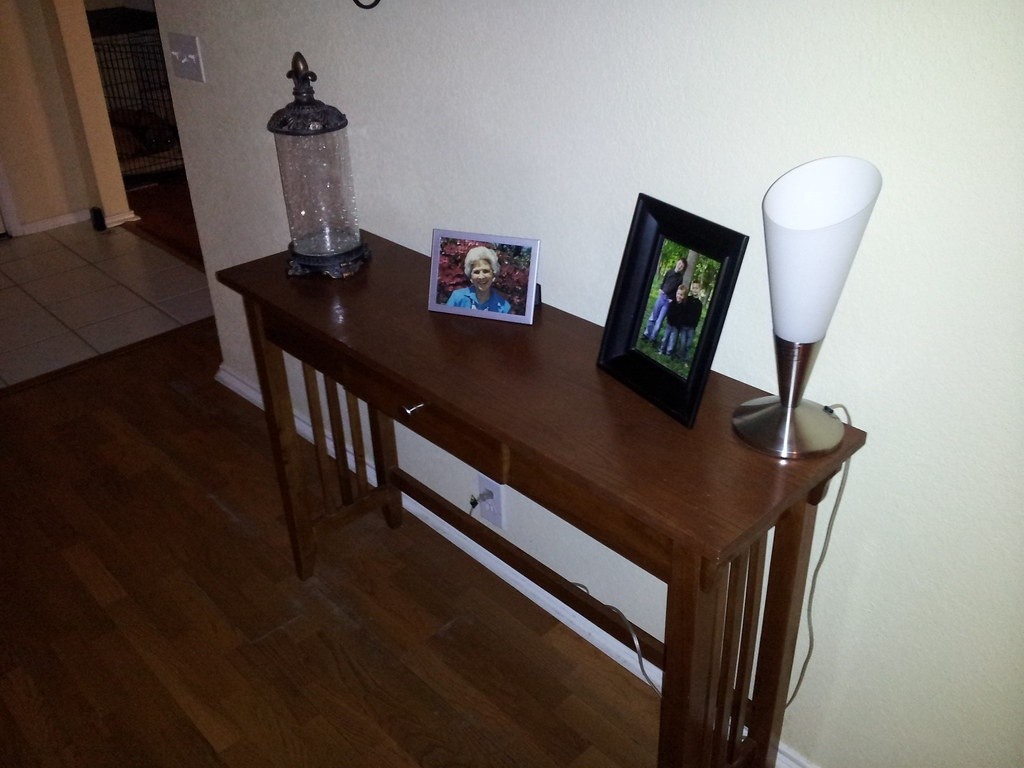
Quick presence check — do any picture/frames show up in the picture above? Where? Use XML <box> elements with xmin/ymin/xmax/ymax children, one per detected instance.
<box><xmin>428</xmin><ymin>228</ymin><xmax>540</xmax><ymax>324</ymax></box>
<box><xmin>595</xmin><ymin>193</ymin><xmax>750</xmax><ymax>430</ymax></box>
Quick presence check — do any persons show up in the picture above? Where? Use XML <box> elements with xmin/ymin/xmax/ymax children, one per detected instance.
<box><xmin>447</xmin><ymin>246</ymin><xmax>511</xmax><ymax>314</ymax></box>
<box><xmin>643</xmin><ymin>257</ymin><xmax>704</xmax><ymax>362</ymax></box>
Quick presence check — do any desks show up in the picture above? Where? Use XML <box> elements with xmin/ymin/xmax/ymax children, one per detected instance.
<box><xmin>215</xmin><ymin>229</ymin><xmax>866</xmax><ymax>768</ymax></box>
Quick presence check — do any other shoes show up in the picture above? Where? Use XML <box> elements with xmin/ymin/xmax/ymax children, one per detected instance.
<box><xmin>640</xmin><ymin>333</ymin><xmax>649</xmax><ymax>340</ymax></box>
<box><xmin>649</xmin><ymin>340</ymin><xmax>656</xmax><ymax>347</ymax></box>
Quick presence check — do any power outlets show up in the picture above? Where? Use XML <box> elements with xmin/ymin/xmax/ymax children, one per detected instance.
<box><xmin>478</xmin><ymin>474</ymin><xmax>502</xmax><ymax>529</ymax></box>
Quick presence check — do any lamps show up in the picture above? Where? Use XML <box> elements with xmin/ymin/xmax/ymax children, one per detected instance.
<box><xmin>731</xmin><ymin>156</ymin><xmax>883</xmax><ymax>457</ymax></box>
